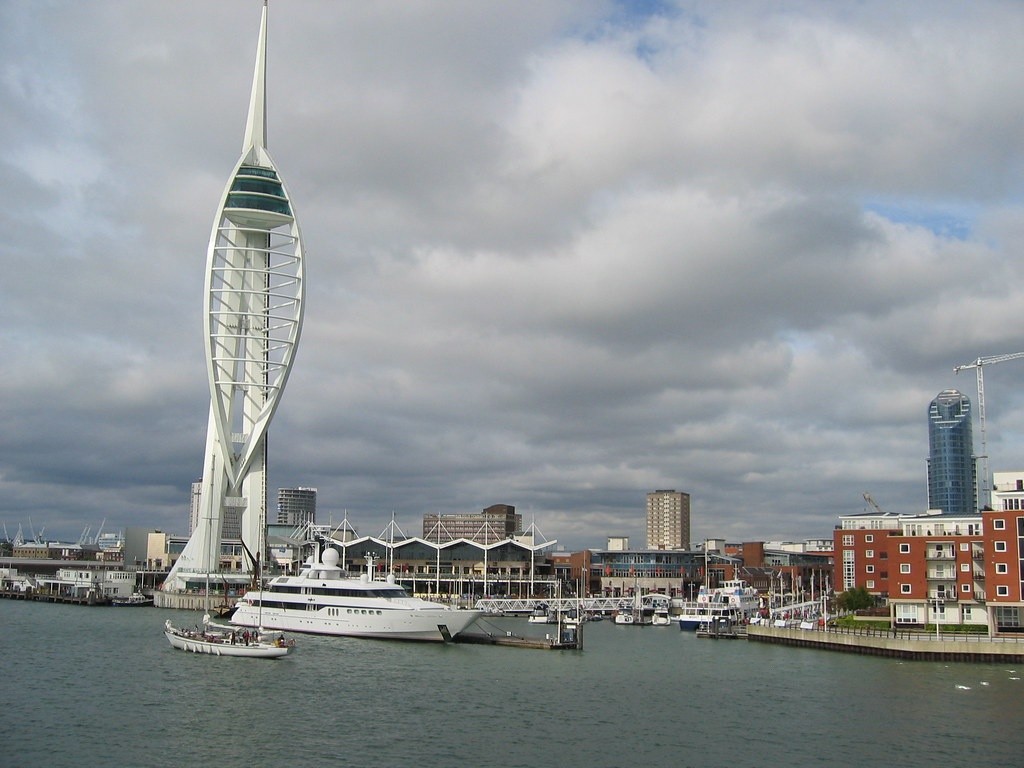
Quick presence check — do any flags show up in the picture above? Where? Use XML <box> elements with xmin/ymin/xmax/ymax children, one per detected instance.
<box><xmin>606</xmin><ymin>564</ymin><xmax>704</xmax><ymax>576</ymax></box>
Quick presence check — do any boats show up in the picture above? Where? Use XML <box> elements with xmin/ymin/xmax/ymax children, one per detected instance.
<box><xmin>163</xmin><ymin>620</ymin><xmax>292</xmax><ymax>658</ymax></box>
<box><xmin>203</xmin><ymin>614</ymin><xmax>284</xmax><ymax>642</ymax></box>
<box><xmin>590</xmin><ymin>613</ymin><xmax>601</xmax><ymax>621</ymax></box>
<box><xmin>651</xmin><ymin>608</ymin><xmax>670</xmax><ymax>626</ymax></box>
<box><xmin>112</xmin><ymin>590</ymin><xmax>154</xmax><ymax>607</ymax></box>
<box><xmin>214</xmin><ymin>548</ymin><xmax>485</xmax><ymax>642</ymax></box>
<box><xmin>528</xmin><ymin>603</ymin><xmax>550</xmax><ymax>624</ymax></box>
<box><xmin>562</xmin><ymin>608</ymin><xmax>587</xmax><ymax>626</ymax></box>
<box><xmin>615</xmin><ymin>608</ymin><xmax>633</xmax><ymax>625</ymax></box>
<box><xmin>679</xmin><ymin>537</ymin><xmax>760</xmax><ymax>631</ymax></box>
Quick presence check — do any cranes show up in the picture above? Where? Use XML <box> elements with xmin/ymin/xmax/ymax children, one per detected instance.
<box><xmin>953</xmin><ymin>351</ymin><xmax>1024</xmax><ymax>510</ymax></box>
<box><xmin>861</xmin><ymin>491</ymin><xmax>878</xmax><ymax>512</ymax></box>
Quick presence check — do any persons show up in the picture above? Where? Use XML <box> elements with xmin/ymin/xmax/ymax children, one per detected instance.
<box><xmin>181</xmin><ymin>625</ymin><xmax>286</xmax><ymax>648</ymax></box>
